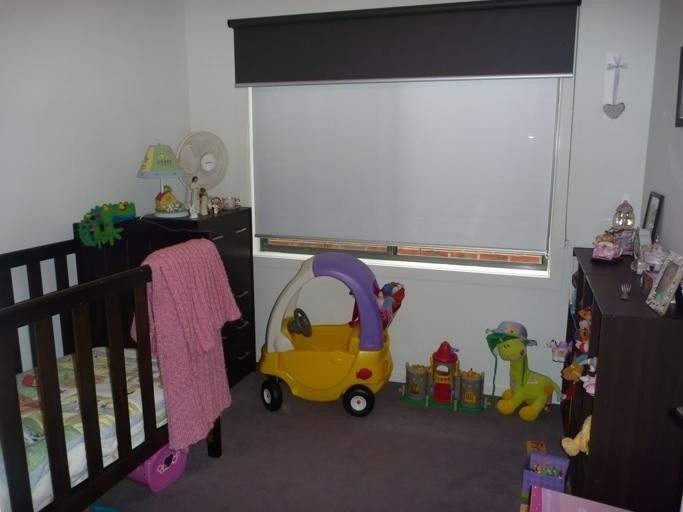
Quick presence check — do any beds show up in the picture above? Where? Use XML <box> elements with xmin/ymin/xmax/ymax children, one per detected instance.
<box><xmin>0</xmin><ymin>219</ymin><xmax>223</xmax><ymax>511</ymax></box>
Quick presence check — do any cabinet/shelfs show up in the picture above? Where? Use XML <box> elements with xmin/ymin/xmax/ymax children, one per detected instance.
<box><xmin>562</xmin><ymin>247</ymin><xmax>683</xmax><ymax>512</ymax></box>
<box><xmin>71</xmin><ymin>205</ymin><xmax>257</xmax><ymax>390</ymax></box>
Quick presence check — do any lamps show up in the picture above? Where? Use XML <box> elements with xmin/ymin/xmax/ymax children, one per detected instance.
<box><xmin>135</xmin><ymin>143</ymin><xmax>184</xmax><ymax>215</ymax></box>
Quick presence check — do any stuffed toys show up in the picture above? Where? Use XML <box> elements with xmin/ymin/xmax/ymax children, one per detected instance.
<box><xmin>561</xmin><ymin>414</ymin><xmax>593</xmax><ymax>455</ymax></box>
<box><xmin>487</xmin><ymin>319</ymin><xmax>563</xmax><ymax>422</ymax></box>
<box><xmin>564</xmin><ymin>302</ymin><xmax>597</xmax><ymax>404</ymax></box>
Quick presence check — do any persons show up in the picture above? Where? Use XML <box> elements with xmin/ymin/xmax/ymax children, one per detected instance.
<box><xmin>191</xmin><ymin>175</ymin><xmax>199</xmax><ymax>216</ymax></box>
<box><xmin>199</xmin><ymin>186</ymin><xmax>209</xmax><ymax>217</ymax></box>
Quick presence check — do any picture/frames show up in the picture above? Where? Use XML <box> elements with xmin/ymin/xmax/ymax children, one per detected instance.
<box><xmin>642</xmin><ymin>190</ymin><xmax>665</xmax><ymax>244</ymax></box>
<box><xmin>675</xmin><ymin>45</ymin><xmax>683</xmax><ymax>129</ymax></box>
<box><xmin>644</xmin><ymin>249</ymin><xmax>683</xmax><ymax>318</ymax></box>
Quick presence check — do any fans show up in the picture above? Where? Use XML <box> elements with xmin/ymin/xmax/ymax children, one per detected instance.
<box><xmin>175</xmin><ymin>130</ymin><xmax>230</xmax><ymax>210</ymax></box>
